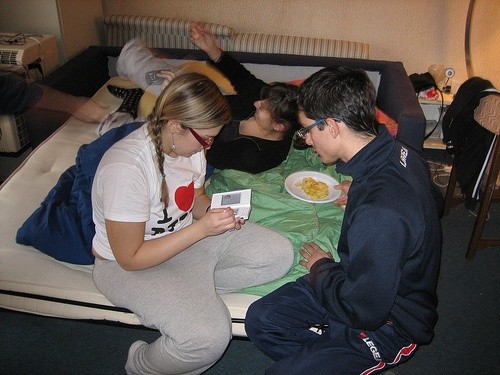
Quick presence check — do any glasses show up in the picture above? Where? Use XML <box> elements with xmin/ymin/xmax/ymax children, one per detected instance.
<box><xmin>189</xmin><ymin>126</ymin><xmax>214</xmax><ymax>150</ymax></box>
<box><xmin>297</xmin><ymin>117</ymin><xmax>341</xmax><ymax>139</ymax></box>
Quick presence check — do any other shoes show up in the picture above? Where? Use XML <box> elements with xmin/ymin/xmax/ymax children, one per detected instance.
<box><xmin>126</xmin><ymin>339</ymin><xmax>150</xmax><ymax>375</ymax></box>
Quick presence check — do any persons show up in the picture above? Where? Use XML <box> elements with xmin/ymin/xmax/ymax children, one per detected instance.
<box><xmin>96</xmin><ymin>20</ymin><xmax>298</xmax><ymax>174</ymax></box>
<box><xmin>243</xmin><ymin>66</ymin><xmax>444</xmax><ymax>375</ymax></box>
<box><xmin>90</xmin><ymin>72</ymin><xmax>295</xmax><ymax>375</ymax></box>
<box><xmin>0</xmin><ymin>69</ymin><xmax>112</xmax><ymax>124</ymax></box>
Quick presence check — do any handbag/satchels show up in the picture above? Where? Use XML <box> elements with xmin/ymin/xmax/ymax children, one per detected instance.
<box><xmin>408</xmin><ymin>72</ymin><xmax>435</xmax><ymax>93</ymax></box>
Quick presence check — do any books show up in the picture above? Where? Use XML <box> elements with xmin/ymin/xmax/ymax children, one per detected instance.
<box><xmin>211</xmin><ymin>189</ymin><xmax>253</xmax><ymax>220</ymax></box>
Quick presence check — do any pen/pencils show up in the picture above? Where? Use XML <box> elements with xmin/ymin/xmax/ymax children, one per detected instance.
<box><xmin>223</xmin><ymin>207</ymin><xmax>231</xmax><ymax>213</ymax></box>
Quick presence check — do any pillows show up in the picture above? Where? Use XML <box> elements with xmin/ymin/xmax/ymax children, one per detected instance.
<box><xmin>288</xmin><ymin>79</ymin><xmax>399</xmax><ymax>139</ymax></box>
<box><xmin>136</xmin><ymin>62</ymin><xmax>238</xmax><ymax>122</ymax></box>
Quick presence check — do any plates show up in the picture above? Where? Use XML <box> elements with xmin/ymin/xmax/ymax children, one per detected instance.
<box><xmin>284</xmin><ymin>170</ymin><xmax>342</xmax><ymax>203</ymax></box>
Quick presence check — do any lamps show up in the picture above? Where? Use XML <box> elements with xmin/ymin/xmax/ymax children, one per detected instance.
<box><xmin>439</xmin><ymin>68</ymin><xmax>455</xmax><ymax>92</ymax></box>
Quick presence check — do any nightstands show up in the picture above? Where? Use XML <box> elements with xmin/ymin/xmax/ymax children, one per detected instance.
<box><xmin>417</xmin><ymin>99</ymin><xmax>451</xmax><ymax>149</ymax></box>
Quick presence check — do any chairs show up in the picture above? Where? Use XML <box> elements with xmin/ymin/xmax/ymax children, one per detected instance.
<box><xmin>442</xmin><ymin>75</ymin><xmax>500</xmax><ymax>261</ymax></box>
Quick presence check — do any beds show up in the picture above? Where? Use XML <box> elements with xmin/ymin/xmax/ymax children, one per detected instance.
<box><xmin>0</xmin><ymin>16</ymin><xmax>427</xmax><ymax>339</ymax></box>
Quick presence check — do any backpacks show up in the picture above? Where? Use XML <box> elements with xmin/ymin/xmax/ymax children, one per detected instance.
<box><xmin>442</xmin><ymin>75</ymin><xmax>500</xmax><ymax>172</ymax></box>
